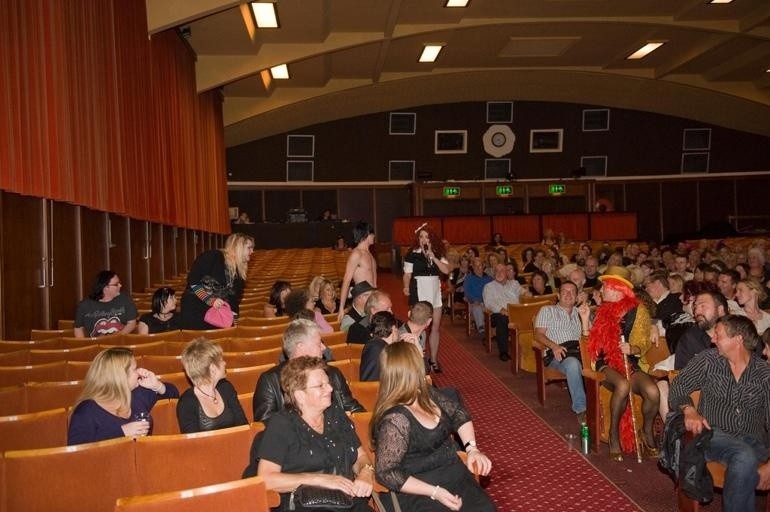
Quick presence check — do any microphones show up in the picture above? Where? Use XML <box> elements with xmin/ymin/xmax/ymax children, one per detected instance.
<box><xmin>425</xmin><ymin>243</ymin><xmax>434</xmax><ymax>266</ymax></box>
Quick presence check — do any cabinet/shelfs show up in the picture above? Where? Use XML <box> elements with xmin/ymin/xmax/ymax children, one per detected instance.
<box><xmin>128</xmin><ymin>216</ymin><xmax>164</xmax><ymax>293</ymax></box>
<box><xmin>216</xmin><ymin>234</ymin><xmax>223</xmax><ymax>249</ymax></box>
<box><xmin>79</xmin><ymin>206</ymin><xmax>131</xmax><ymax>298</ymax></box>
<box><xmin>203</xmin><ymin>232</ymin><xmax>217</xmax><ymax>251</ymax></box>
<box><xmin>162</xmin><ymin>224</ymin><xmax>187</xmax><ymax>279</ymax></box>
<box><xmin>185</xmin><ymin>229</ymin><xmax>204</xmax><ymax>273</ymax></box>
<box><xmin>0</xmin><ymin>190</ymin><xmax>82</xmax><ymax>339</ymax></box>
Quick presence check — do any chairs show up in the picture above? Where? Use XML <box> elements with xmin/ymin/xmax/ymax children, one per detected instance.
<box><xmin>31</xmin><ymin>327</ymin><xmax>87</xmax><ymax>341</ymax></box>
<box><xmin>66</xmin><ymin>397</ymin><xmax>169</xmax><ymax>436</ymax></box>
<box><xmin>113</xmin><ymin>474</ymin><xmax>268</xmax><ymax>512</ymax></box>
<box><xmin>531</xmin><ymin>315</ymin><xmax>567</xmax><ymax>408</ymax></box>
<box><xmin>1</xmin><ymin>339</ymin><xmax>59</xmax><ymax>353</ymax></box>
<box><xmin>350</xmin><ymin>375</ymin><xmax>432</xmax><ymax>412</ymax></box>
<box><xmin>229</xmin><ymin>346</ymin><xmax>283</xmax><ymax>367</ymax></box>
<box><xmin>348</xmin><ymin>342</ymin><xmax>365</xmax><ymax>358</ymax></box>
<box><xmin>577</xmin><ymin>336</ymin><xmax>642</xmax><ymax>454</ymax></box>
<box><xmin>239</xmin><ymin>309</ymin><xmax>265</xmax><ymax>316</ymax></box>
<box><xmin>228</xmin><ymin>334</ymin><xmax>286</xmax><ymax>352</ymax></box>
<box><xmin>247</xmin><ymin>315</ymin><xmax>289</xmax><ymax>325</ymax></box>
<box><xmin>520</xmin><ymin>294</ymin><xmax>557</xmax><ymax>304</ymax></box>
<box><xmin>134</xmin><ymin>423</ymin><xmax>251</xmax><ymax>491</ymax></box>
<box><xmin>0</xmin><ymin>407</ymin><xmax>66</xmax><ymax>450</ymax></box>
<box><xmin>351</xmin><ymin>410</ymin><xmax>390</xmax><ymax>495</ymax></box>
<box><xmin>29</xmin><ymin>344</ymin><xmax>98</xmax><ymax>364</ymax></box>
<box><xmin>66</xmin><ymin>356</ymin><xmax>141</xmax><ymax>380</ymax></box>
<box><xmin>62</xmin><ymin>334</ymin><xmax>123</xmax><ymax>349</ymax></box>
<box><xmin>221</xmin><ymin>350</ymin><xmax>230</xmax><ymax>371</ymax></box>
<box><xmin>166</xmin><ymin>337</ymin><xmax>227</xmax><ymax>356</ymax></box>
<box><xmin>250</xmin><ymin>410</ymin><xmax>351</xmax><ymax>443</ymax></box>
<box><xmin>0</xmin><ymin>382</ymin><xmax>27</xmax><ymax>415</ymax></box>
<box><xmin>328</xmin><ymin>342</ymin><xmax>348</xmax><ymax>361</ymax></box>
<box><xmin>100</xmin><ymin>340</ymin><xmax>164</xmax><ymax>359</ymax></box>
<box><xmin>646</xmin><ymin>336</ymin><xmax>669</xmax><ymax>367</ymax></box>
<box><xmin>323</xmin><ymin>311</ymin><xmax>337</xmax><ymax>322</ymax></box>
<box><xmin>238</xmin><ymin>246</ymin><xmax>353</xmax><ymax>295</ymax></box>
<box><xmin>26</xmin><ymin>379</ymin><xmax>85</xmax><ymax>413</ymax></box>
<box><xmin>168</xmin><ymin>399</ymin><xmax>180</xmax><ymax>435</ymax></box>
<box><xmin>241</xmin><ymin>296</ymin><xmax>265</xmax><ymax>303</ymax></box>
<box><xmin>351</xmin><ymin>357</ymin><xmax>362</xmax><ymax>383</ymax></box>
<box><xmin>323</xmin><ymin>330</ymin><xmax>347</xmax><ymax>344</ymax></box>
<box><xmin>1</xmin><ymin>349</ymin><xmax>29</xmax><ymax>367</ymax></box>
<box><xmin>126</xmin><ymin>329</ymin><xmax>181</xmax><ymax>345</ymax></box>
<box><xmin>226</xmin><ymin>363</ymin><xmax>276</xmax><ymax>395</ymax></box>
<box><xmin>133</xmin><ymin>273</ymin><xmax>186</xmax><ymax>321</ymax></box>
<box><xmin>507</xmin><ymin>300</ymin><xmax>552</xmax><ymax>374</ymax></box>
<box><xmin>236</xmin><ymin>390</ymin><xmax>254</xmax><ymax>426</ymax></box>
<box><xmin>239</xmin><ymin>302</ymin><xmax>264</xmax><ymax>310</ymax></box>
<box><xmin>329</xmin><ymin>320</ymin><xmax>342</xmax><ymax>329</ymax></box>
<box><xmin>157</xmin><ymin>370</ymin><xmax>192</xmax><ymax>398</ymax></box>
<box><xmin>3</xmin><ymin>435</ymin><xmax>136</xmax><ymax>512</ymax></box>
<box><xmin>707</xmin><ymin>461</ymin><xmax>766</xmax><ymax>490</ymax></box>
<box><xmin>181</xmin><ymin>327</ymin><xmax>237</xmax><ymax>339</ymax></box>
<box><xmin>237</xmin><ymin>323</ymin><xmax>288</xmax><ymax>337</ymax></box>
<box><xmin>328</xmin><ymin>359</ymin><xmax>351</xmax><ymax>384</ymax></box>
<box><xmin>142</xmin><ymin>353</ymin><xmax>185</xmax><ymax>373</ymax></box>
<box><xmin>1</xmin><ymin>360</ymin><xmax>66</xmax><ymax>385</ymax></box>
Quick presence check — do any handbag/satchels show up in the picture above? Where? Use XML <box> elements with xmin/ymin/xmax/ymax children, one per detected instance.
<box><xmin>292</xmin><ymin>485</ymin><xmax>355</xmax><ymax>512</ymax></box>
<box><xmin>204</xmin><ymin>302</ymin><xmax>234</xmax><ymax>329</ymax></box>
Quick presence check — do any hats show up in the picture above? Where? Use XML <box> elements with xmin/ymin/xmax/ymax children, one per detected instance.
<box><xmin>598</xmin><ymin>266</ymin><xmax>635</xmax><ymax>291</ymax></box>
<box><xmin>348</xmin><ymin>280</ymin><xmax>378</xmax><ymax>302</ymax></box>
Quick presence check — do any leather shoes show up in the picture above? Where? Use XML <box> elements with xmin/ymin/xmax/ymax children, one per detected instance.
<box><xmin>498</xmin><ymin>352</ymin><xmax>508</xmax><ymax>362</ymax></box>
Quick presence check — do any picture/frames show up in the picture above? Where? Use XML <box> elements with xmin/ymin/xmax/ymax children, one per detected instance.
<box><xmin>683</xmin><ymin>128</ymin><xmax>711</xmax><ymax>151</ymax></box>
<box><xmin>485</xmin><ymin>159</ymin><xmax>511</xmax><ymax>180</ymax></box>
<box><xmin>580</xmin><ymin>156</ymin><xmax>607</xmax><ymax>176</ymax></box>
<box><xmin>286</xmin><ymin>161</ymin><xmax>314</xmax><ymax>182</ymax></box>
<box><xmin>529</xmin><ymin>129</ymin><xmax>563</xmax><ymax>153</ymax></box>
<box><xmin>681</xmin><ymin>152</ymin><xmax>709</xmax><ymax>175</ymax></box>
<box><xmin>582</xmin><ymin>109</ymin><xmax>609</xmax><ymax>132</ymax></box>
<box><xmin>486</xmin><ymin>102</ymin><xmax>513</xmax><ymax>123</ymax></box>
<box><xmin>389</xmin><ymin>112</ymin><xmax>416</xmax><ymax>135</ymax></box>
<box><xmin>388</xmin><ymin>161</ymin><xmax>415</xmax><ymax>181</ymax></box>
<box><xmin>434</xmin><ymin>131</ymin><xmax>467</xmax><ymax>154</ymax></box>
<box><xmin>287</xmin><ymin>135</ymin><xmax>314</xmax><ymax>158</ymax></box>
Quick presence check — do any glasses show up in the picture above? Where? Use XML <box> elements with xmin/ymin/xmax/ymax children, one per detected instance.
<box><xmin>106</xmin><ymin>282</ymin><xmax>120</xmax><ymax>287</ymax></box>
<box><xmin>298</xmin><ymin>381</ymin><xmax>331</xmax><ymax>392</ymax></box>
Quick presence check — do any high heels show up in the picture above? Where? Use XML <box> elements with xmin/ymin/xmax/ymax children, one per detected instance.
<box><xmin>427</xmin><ymin>358</ymin><xmax>441</xmax><ymax>374</ymax></box>
<box><xmin>638</xmin><ymin>430</ymin><xmax>662</xmax><ymax>456</ymax></box>
<box><xmin>607</xmin><ymin>437</ymin><xmax>625</xmax><ymax>462</ymax></box>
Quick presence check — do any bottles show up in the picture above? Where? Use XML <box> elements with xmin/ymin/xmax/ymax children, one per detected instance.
<box><xmin>581</xmin><ymin>427</ymin><xmax>590</xmax><ymax>454</ymax></box>
<box><xmin>580</xmin><ymin>422</ymin><xmax>589</xmax><ymax>447</ymax></box>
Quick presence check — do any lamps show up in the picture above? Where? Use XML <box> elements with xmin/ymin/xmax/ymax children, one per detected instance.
<box><xmin>270</xmin><ymin>63</ymin><xmax>290</xmax><ymax>80</ymax></box>
<box><xmin>250</xmin><ymin>2</ymin><xmax>280</xmax><ymax>29</ymax></box>
<box><xmin>624</xmin><ymin>40</ymin><xmax>667</xmax><ymax>61</ymax></box>
<box><xmin>708</xmin><ymin>0</ymin><xmax>735</xmax><ymax>4</ymax></box>
<box><xmin>445</xmin><ymin>0</ymin><xmax>472</xmax><ymax>8</ymax></box>
<box><xmin>416</xmin><ymin>44</ymin><xmax>445</xmax><ymax>63</ymax></box>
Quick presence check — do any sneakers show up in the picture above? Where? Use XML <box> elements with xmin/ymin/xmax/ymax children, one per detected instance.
<box><xmin>578</xmin><ymin>412</ymin><xmax>587</xmax><ymax>425</ymax></box>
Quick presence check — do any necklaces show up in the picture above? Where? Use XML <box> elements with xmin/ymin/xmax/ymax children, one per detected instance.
<box><xmin>196</xmin><ymin>384</ymin><xmax>218</xmax><ymax>404</ymax></box>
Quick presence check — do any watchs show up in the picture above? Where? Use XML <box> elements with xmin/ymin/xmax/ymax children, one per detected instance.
<box><xmin>464</xmin><ymin>440</ymin><xmax>476</xmax><ymax>449</ymax></box>
<box><xmin>358</xmin><ymin>463</ymin><xmax>375</xmax><ymax>472</ymax></box>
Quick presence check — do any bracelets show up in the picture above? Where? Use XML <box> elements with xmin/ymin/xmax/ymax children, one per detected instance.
<box><xmin>152</xmin><ymin>381</ymin><xmax>163</xmax><ymax>392</ymax></box>
<box><xmin>430</xmin><ymin>485</ymin><xmax>440</xmax><ymax>501</ymax></box>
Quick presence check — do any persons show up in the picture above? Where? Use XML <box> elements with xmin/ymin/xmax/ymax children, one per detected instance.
<box><xmin>673</xmin><ymin>289</ymin><xmax>729</xmax><ymax>371</ymax></box>
<box><xmin>505</xmin><ymin>262</ymin><xmax>527</xmax><ymax>286</ymax></box>
<box><xmin>634</xmin><ymin>286</ymin><xmax>666</xmax><ymax>347</ymax></box>
<box><xmin>311</xmin><ymin>210</ymin><xmax>349</xmax><ymax>250</ymax></box>
<box><xmin>368</xmin><ymin>342</ymin><xmax>498</xmax><ymax>512</ymax></box>
<box><xmin>256</xmin><ymin>355</ymin><xmax>375</xmax><ymax>511</ymax></box>
<box><xmin>463</xmin><ymin>258</ymin><xmax>493</xmax><ymax>338</ymax></box>
<box><xmin>727</xmin><ymin>279</ymin><xmax>770</xmax><ymax>334</ymax></box>
<box><xmin>656</xmin><ymin>237</ymin><xmax>770</xmax><ymax>296</ymax></box>
<box><xmin>482</xmin><ymin>264</ymin><xmax>528</xmax><ymax>362</ymax></box>
<box><xmin>533</xmin><ymin>281</ymin><xmax>592</xmax><ymax>425</ymax></box>
<box><xmin>359</xmin><ymin>311</ymin><xmax>424</xmax><ymax>381</ymax></box>
<box><xmin>518</xmin><ymin>228</ymin><xmax>585</xmax><ymax>295</ymax></box>
<box><xmin>340</xmin><ymin>280</ymin><xmax>380</xmax><ymax>331</ymax></box>
<box><xmin>668</xmin><ymin>314</ymin><xmax>770</xmax><ymax>512</ymax></box>
<box><xmin>67</xmin><ymin>347</ymin><xmax>179</xmax><ymax>446</ymax></box>
<box><xmin>346</xmin><ymin>291</ymin><xmax>394</xmax><ymax>343</ymax></box>
<box><xmin>73</xmin><ymin>271</ymin><xmax>139</xmax><ymax>338</ymax></box>
<box><xmin>585</xmin><ymin>239</ymin><xmax>655</xmax><ymax>287</ymax></box>
<box><xmin>441</xmin><ymin>238</ymin><xmax>460</xmax><ymax>285</ymax></box>
<box><xmin>138</xmin><ymin>288</ymin><xmax>179</xmax><ymax>335</ymax></box>
<box><xmin>336</xmin><ymin>222</ymin><xmax>377</xmax><ymax>322</ymax></box>
<box><xmin>264</xmin><ymin>275</ymin><xmax>348</xmax><ymax>364</ymax></box>
<box><xmin>402</xmin><ymin>223</ymin><xmax>451</xmax><ymax>374</ymax></box>
<box><xmin>468</xmin><ymin>233</ymin><xmax>508</xmax><ymax>276</ymax></box>
<box><xmin>448</xmin><ymin>255</ymin><xmax>472</xmax><ymax>302</ymax></box>
<box><xmin>180</xmin><ymin>234</ymin><xmax>255</xmax><ymax>331</ymax></box>
<box><xmin>252</xmin><ymin>319</ymin><xmax>368</xmax><ymax>421</ymax></box>
<box><xmin>176</xmin><ymin>339</ymin><xmax>249</xmax><ymax>434</ymax></box>
<box><xmin>644</xmin><ymin>272</ymin><xmax>683</xmax><ymax>339</ymax></box>
<box><xmin>398</xmin><ymin>301</ymin><xmax>434</xmax><ymax>347</ymax></box>
<box><xmin>578</xmin><ymin>265</ymin><xmax>660</xmax><ymax>462</ymax></box>
<box><xmin>759</xmin><ymin>327</ymin><xmax>770</xmax><ymax>362</ymax></box>
